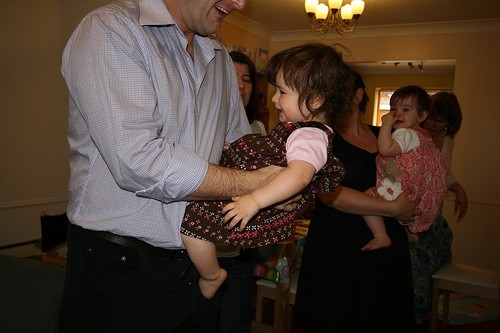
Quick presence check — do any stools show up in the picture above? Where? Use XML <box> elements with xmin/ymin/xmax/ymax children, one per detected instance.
<box><xmin>430</xmin><ymin>263</ymin><xmax>500</xmax><ymax>333</ymax></box>
<box><xmin>251</xmin><ymin>269</ymin><xmax>299</xmax><ymax>333</ymax></box>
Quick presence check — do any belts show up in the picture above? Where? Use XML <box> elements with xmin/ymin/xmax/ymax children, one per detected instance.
<box><xmin>71</xmin><ymin>220</ymin><xmax>185</xmax><ymax>262</ymax></box>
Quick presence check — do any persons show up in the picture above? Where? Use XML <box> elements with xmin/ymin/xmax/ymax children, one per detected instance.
<box><xmin>291</xmin><ymin>68</ymin><xmax>423</xmax><ymax>333</ymax></box>
<box><xmin>215</xmin><ymin>51</ymin><xmax>269</xmax><ymax>333</ymax></box>
<box><xmin>359</xmin><ymin>86</ymin><xmax>450</xmax><ymax>252</ymax></box>
<box><xmin>404</xmin><ymin>91</ymin><xmax>468</xmax><ymax>333</ymax></box>
<box><xmin>179</xmin><ymin>44</ymin><xmax>353</xmax><ymax>301</ymax></box>
<box><xmin>60</xmin><ymin>0</ymin><xmax>301</xmax><ymax>333</ymax></box>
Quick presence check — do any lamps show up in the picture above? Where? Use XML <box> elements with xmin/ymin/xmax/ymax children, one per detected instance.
<box><xmin>304</xmin><ymin>0</ymin><xmax>365</xmax><ymax>37</ymax></box>
